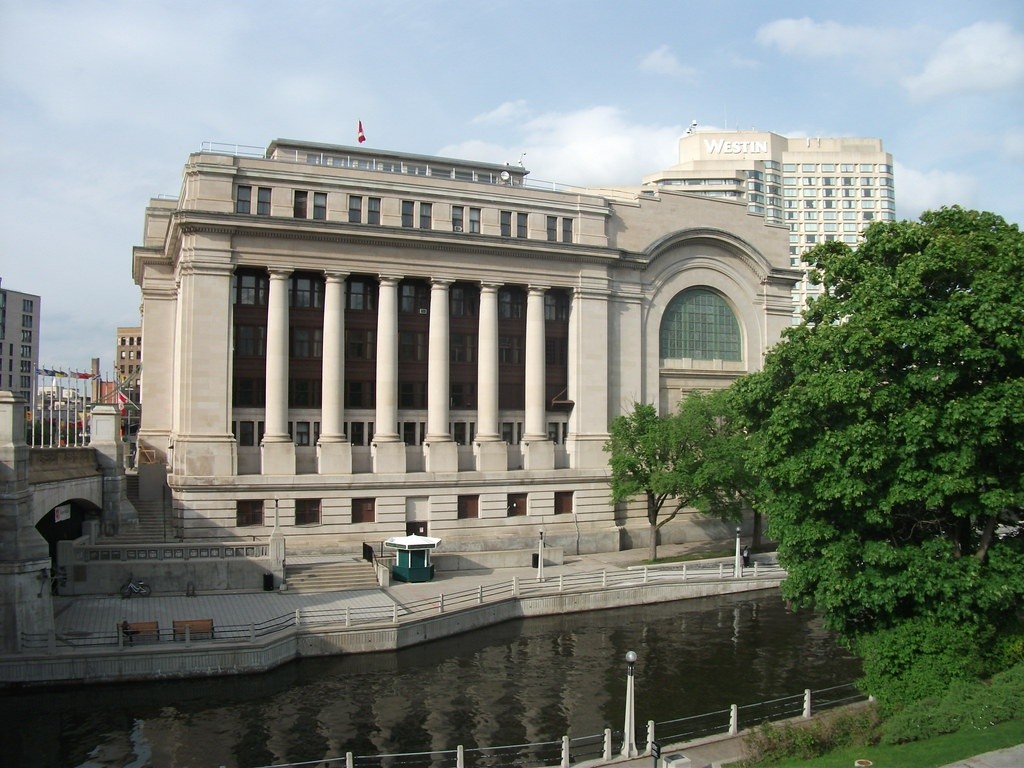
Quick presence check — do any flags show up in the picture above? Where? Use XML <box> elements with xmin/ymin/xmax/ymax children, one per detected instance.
<box><xmin>35</xmin><ymin>367</ymin><xmax>95</xmax><ymax>379</ymax></box>
<box><xmin>116</xmin><ymin>390</ymin><xmax>128</xmax><ymax>416</ymax></box>
<box><xmin>357</xmin><ymin>119</ymin><xmax>366</xmax><ymax>143</ymax></box>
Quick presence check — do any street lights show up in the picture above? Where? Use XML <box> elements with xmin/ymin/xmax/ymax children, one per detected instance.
<box><xmin>732</xmin><ymin>526</ymin><xmax>743</xmax><ymax>580</ymax></box>
<box><xmin>535</xmin><ymin>528</ymin><xmax>546</xmax><ymax>583</ymax></box>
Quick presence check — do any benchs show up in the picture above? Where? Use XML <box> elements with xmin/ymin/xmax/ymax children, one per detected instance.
<box><xmin>173</xmin><ymin>619</ymin><xmax>214</xmax><ymax>639</ymax></box>
<box><xmin>117</xmin><ymin>621</ymin><xmax>160</xmax><ymax>642</ymax></box>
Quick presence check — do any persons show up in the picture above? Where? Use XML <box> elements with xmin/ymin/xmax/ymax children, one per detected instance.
<box><xmin>743</xmin><ymin>546</ymin><xmax>750</xmax><ymax>568</ymax></box>
<box><xmin>121</xmin><ymin>620</ymin><xmax>137</xmax><ymax>647</ymax></box>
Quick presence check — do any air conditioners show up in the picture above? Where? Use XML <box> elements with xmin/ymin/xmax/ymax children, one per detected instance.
<box><xmin>454</xmin><ymin>226</ymin><xmax>463</xmax><ymax>232</ymax></box>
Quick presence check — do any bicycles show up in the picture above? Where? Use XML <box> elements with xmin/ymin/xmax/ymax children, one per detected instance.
<box><xmin>119</xmin><ymin>572</ymin><xmax>152</xmax><ymax>599</ymax></box>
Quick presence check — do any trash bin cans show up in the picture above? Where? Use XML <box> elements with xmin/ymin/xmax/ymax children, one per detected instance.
<box><xmin>262</xmin><ymin>573</ymin><xmax>274</xmax><ymax>591</ymax></box>
<box><xmin>531</xmin><ymin>553</ymin><xmax>539</xmax><ymax>568</ymax></box>
